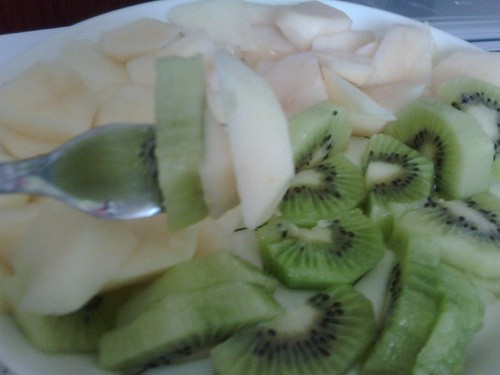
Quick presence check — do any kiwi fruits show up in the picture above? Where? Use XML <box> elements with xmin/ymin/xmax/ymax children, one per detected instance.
<box><xmin>155</xmin><ymin>52</ymin><xmax>210</xmax><ymax>232</ymax></box>
<box><xmin>11</xmin><ymin>76</ymin><xmax>500</xmax><ymax>375</ymax></box>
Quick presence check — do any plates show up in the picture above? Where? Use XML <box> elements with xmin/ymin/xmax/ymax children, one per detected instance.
<box><xmin>2</xmin><ymin>0</ymin><xmax>499</xmax><ymax>375</ymax></box>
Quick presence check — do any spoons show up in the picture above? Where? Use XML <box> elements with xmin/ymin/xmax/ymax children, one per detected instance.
<box><xmin>1</xmin><ymin>122</ymin><xmax>166</xmax><ymax>219</ymax></box>
